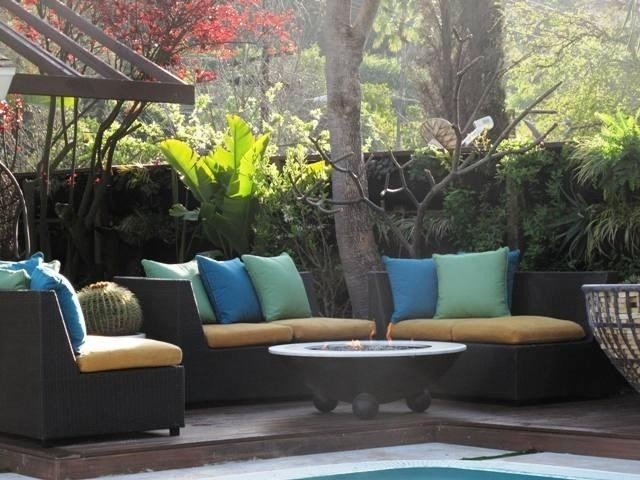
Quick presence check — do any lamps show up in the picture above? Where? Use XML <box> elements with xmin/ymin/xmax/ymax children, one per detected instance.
<box><xmin>0</xmin><ymin>53</ymin><xmax>17</xmax><ymax>102</ymax></box>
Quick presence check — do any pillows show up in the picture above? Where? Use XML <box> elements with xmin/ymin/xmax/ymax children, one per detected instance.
<box><xmin>0</xmin><ymin>251</ymin><xmax>87</xmax><ymax>352</ymax></box>
<box><xmin>386</xmin><ymin>245</ymin><xmax>520</xmax><ymax>322</ymax></box>
<box><xmin>140</xmin><ymin>253</ymin><xmax>314</xmax><ymax>324</ymax></box>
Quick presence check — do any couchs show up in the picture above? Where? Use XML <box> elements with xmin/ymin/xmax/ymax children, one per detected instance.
<box><xmin>0</xmin><ymin>288</ymin><xmax>188</xmax><ymax>448</ymax></box>
<box><xmin>365</xmin><ymin>269</ymin><xmax>618</xmax><ymax>408</ymax></box>
<box><xmin>111</xmin><ymin>271</ymin><xmax>320</xmax><ymax>413</ymax></box>
